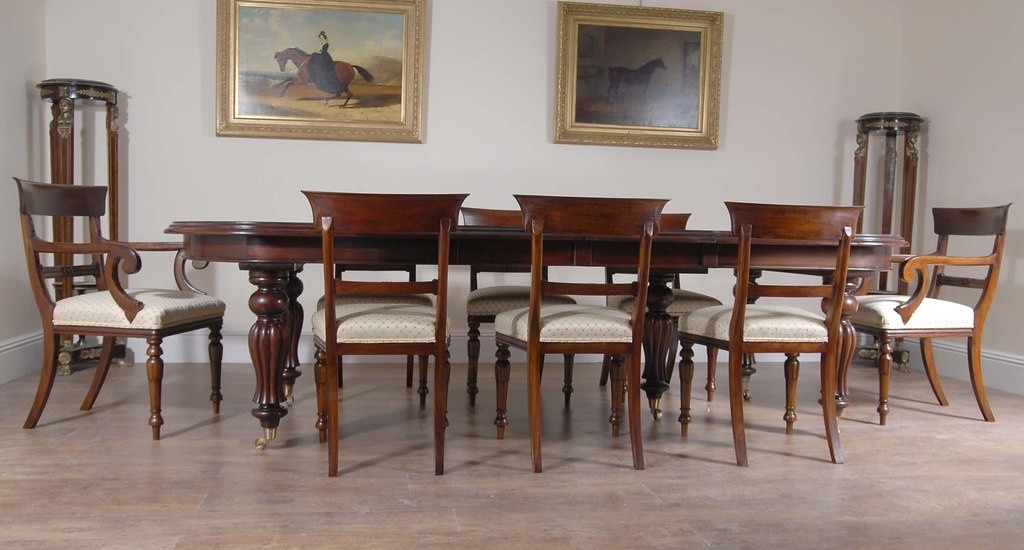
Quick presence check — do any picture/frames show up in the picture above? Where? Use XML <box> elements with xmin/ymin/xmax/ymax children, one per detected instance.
<box><xmin>554</xmin><ymin>1</ymin><xmax>725</xmax><ymax>152</ymax></box>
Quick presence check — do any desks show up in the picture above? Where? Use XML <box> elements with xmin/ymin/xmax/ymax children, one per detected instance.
<box><xmin>164</xmin><ymin>219</ymin><xmax>909</xmax><ymax>451</ymax></box>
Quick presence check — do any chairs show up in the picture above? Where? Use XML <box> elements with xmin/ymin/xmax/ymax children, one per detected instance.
<box><xmin>678</xmin><ymin>201</ymin><xmax>866</xmax><ymax>469</ymax></box>
<box><xmin>819</xmin><ymin>202</ymin><xmax>1011</xmax><ymax>423</ymax></box>
<box><xmin>302</xmin><ymin>189</ymin><xmax>471</xmax><ymax>478</ymax></box>
<box><xmin>459</xmin><ymin>206</ymin><xmax>579</xmax><ymax>408</ymax></box>
<box><xmin>216</xmin><ymin>0</ymin><xmax>432</xmax><ymax>142</ymax></box>
<box><xmin>600</xmin><ymin>211</ymin><xmax>724</xmax><ymax>401</ymax></box>
<box><xmin>12</xmin><ymin>176</ymin><xmax>226</xmax><ymax>441</ymax></box>
<box><xmin>334</xmin><ymin>263</ymin><xmax>433</xmax><ymax>407</ymax></box>
<box><xmin>493</xmin><ymin>193</ymin><xmax>672</xmax><ymax>474</ymax></box>
